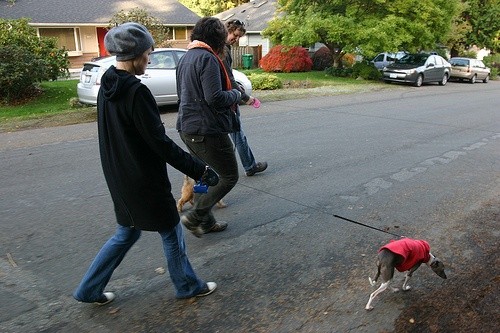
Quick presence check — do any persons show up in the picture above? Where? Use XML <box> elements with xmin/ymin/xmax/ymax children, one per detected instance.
<box><xmin>72</xmin><ymin>23</ymin><xmax>218</xmax><ymax>305</ymax></box>
<box><xmin>217</xmin><ymin>19</ymin><xmax>269</xmax><ymax>177</ymax></box>
<box><xmin>174</xmin><ymin>16</ymin><xmax>242</xmax><ymax>238</ymax></box>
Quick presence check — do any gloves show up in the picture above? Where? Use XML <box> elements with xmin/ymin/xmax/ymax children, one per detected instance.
<box><xmin>204</xmin><ymin>168</ymin><xmax>220</xmax><ymax>187</ymax></box>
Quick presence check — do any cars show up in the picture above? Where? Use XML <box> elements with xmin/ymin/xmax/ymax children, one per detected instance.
<box><xmin>76</xmin><ymin>47</ymin><xmax>252</xmax><ymax>111</ymax></box>
<box><xmin>382</xmin><ymin>53</ymin><xmax>452</xmax><ymax>87</ymax></box>
<box><xmin>448</xmin><ymin>57</ymin><xmax>492</xmax><ymax>84</ymax></box>
<box><xmin>370</xmin><ymin>51</ymin><xmax>410</xmax><ymax>73</ymax></box>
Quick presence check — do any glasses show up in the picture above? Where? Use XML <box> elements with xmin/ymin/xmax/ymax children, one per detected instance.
<box><xmin>234</xmin><ymin>20</ymin><xmax>246</xmax><ymax>26</ymax></box>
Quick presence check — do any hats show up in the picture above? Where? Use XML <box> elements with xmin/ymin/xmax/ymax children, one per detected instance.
<box><xmin>103</xmin><ymin>22</ymin><xmax>155</xmax><ymax>62</ymax></box>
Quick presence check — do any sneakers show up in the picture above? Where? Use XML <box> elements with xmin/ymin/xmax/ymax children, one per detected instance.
<box><xmin>201</xmin><ymin>221</ymin><xmax>228</xmax><ymax>233</ymax></box>
<box><xmin>181</xmin><ymin>213</ymin><xmax>205</xmax><ymax>239</ymax></box>
<box><xmin>246</xmin><ymin>161</ymin><xmax>268</xmax><ymax>176</ymax></box>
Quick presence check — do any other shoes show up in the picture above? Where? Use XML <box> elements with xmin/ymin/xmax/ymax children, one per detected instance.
<box><xmin>193</xmin><ymin>281</ymin><xmax>218</xmax><ymax>296</ymax></box>
<box><xmin>74</xmin><ymin>291</ymin><xmax>115</xmax><ymax>305</ymax></box>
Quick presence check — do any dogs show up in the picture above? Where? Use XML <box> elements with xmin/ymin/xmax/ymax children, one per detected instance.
<box><xmin>365</xmin><ymin>238</ymin><xmax>447</xmax><ymax>311</ymax></box>
<box><xmin>177</xmin><ymin>175</ymin><xmax>227</xmax><ymax>213</ymax></box>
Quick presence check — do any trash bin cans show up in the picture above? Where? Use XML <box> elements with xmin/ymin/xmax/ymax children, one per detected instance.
<box><xmin>241</xmin><ymin>53</ymin><xmax>253</xmax><ymax>69</ymax></box>
